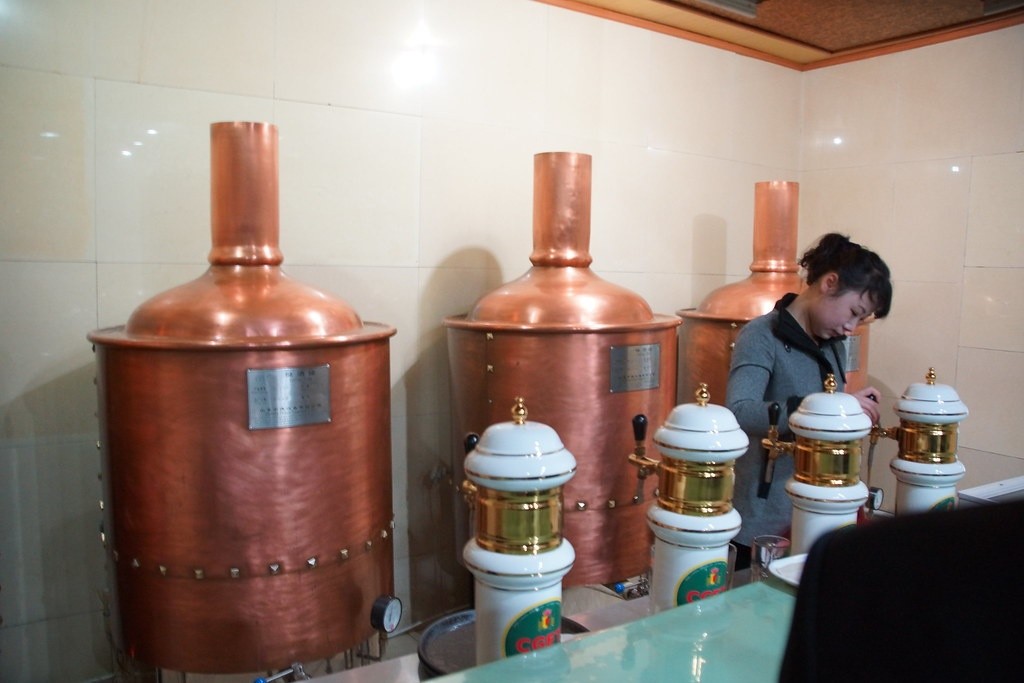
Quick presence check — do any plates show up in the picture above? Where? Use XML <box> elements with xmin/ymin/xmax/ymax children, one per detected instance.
<box><xmin>771</xmin><ymin>550</ymin><xmax>812</xmax><ymax>591</ymax></box>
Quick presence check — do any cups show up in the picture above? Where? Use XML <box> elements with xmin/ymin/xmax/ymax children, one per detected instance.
<box><xmin>748</xmin><ymin>534</ymin><xmax>790</xmax><ymax>588</ymax></box>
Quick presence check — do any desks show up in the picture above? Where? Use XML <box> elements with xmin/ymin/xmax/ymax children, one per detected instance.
<box><xmin>417</xmin><ymin>557</ymin><xmax>810</xmax><ymax>683</ymax></box>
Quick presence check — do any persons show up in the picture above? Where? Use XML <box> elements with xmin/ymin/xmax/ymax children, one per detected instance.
<box><xmin>727</xmin><ymin>232</ymin><xmax>892</xmax><ymax>574</ymax></box>
